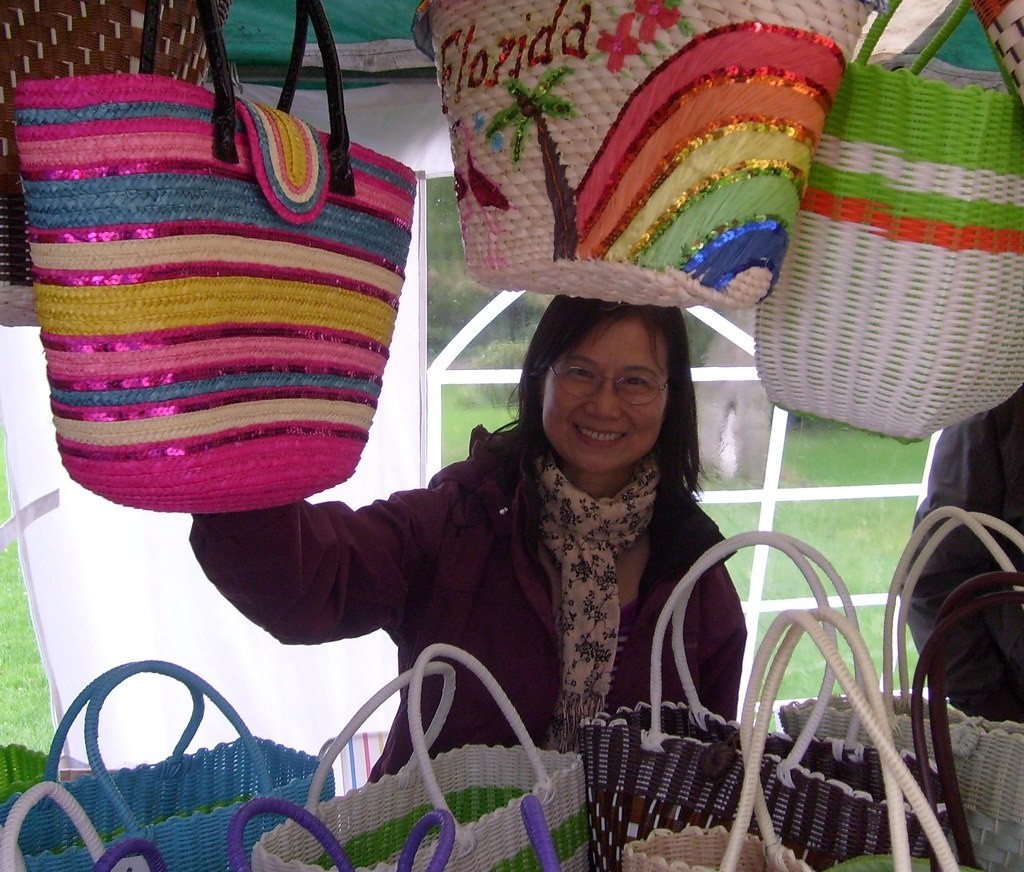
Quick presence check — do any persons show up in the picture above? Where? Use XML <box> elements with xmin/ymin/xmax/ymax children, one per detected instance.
<box><xmin>188</xmin><ymin>294</ymin><xmax>748</xmax><ymax>784</ymax></box>
<box><xmin>901</xmin><ymin>385</ymin><xmax>1024</xmax><ymax>721</ymax></box>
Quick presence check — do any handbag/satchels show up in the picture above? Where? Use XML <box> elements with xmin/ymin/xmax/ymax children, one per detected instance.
<box><xmin>754</xmin><ymin>0</ymin><xmax>1024</xmax><ymax>444</ymax></box>
<box><xmin>0</xmin><ymin>0</ymin><xmax>233</xmax><ymax>328</ymax></box>
<box><xmin>428</xmin><ymin>0</ymin><xmax>877</xmax><ymax>312</ymax></box>
<box><xmin>14</xmin><ymin>0</ymin><xmax>415</xmax><ymax>514</ymax></box>
<box><xmin>0</xmin><ymin>503</ymin><xmax>1024</xmax><ymax>872</ymax></box>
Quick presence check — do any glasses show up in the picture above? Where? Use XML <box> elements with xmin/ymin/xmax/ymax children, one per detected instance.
<box><xmin>549</xmin><ymin>363</ymin><xmax>667</xmax><ymax>406</ymax></box>
